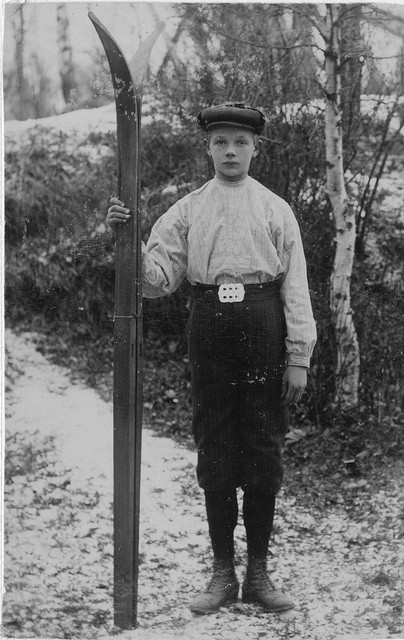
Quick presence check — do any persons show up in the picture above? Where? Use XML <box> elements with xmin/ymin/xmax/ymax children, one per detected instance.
<box><xmin>105</xmin><ymin>105</ymin><xmax>320</xmax><ymax>614</ymax></box>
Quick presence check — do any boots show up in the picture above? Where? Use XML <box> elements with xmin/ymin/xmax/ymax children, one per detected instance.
<box><xmin>188</xmin><ymin>559</ymin><xmax>239</xmax><ymax>614</ymax></box>
<box><xmin>241</xmin><ymin>556</ymin><xmax>294</xmax><ymax>613</ymax></box>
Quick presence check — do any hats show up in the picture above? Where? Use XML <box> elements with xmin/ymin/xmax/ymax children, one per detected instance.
<box><xmin>195</xmin><ymin>102</ymin><xmax>266</xmax><ymax>134</ymax></box>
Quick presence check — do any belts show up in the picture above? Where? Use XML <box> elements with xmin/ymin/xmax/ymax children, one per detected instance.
<box><xmin>194</xmin><ymin>279</ymin><xmax>280</xmax><ymax>304</ymax></box>
